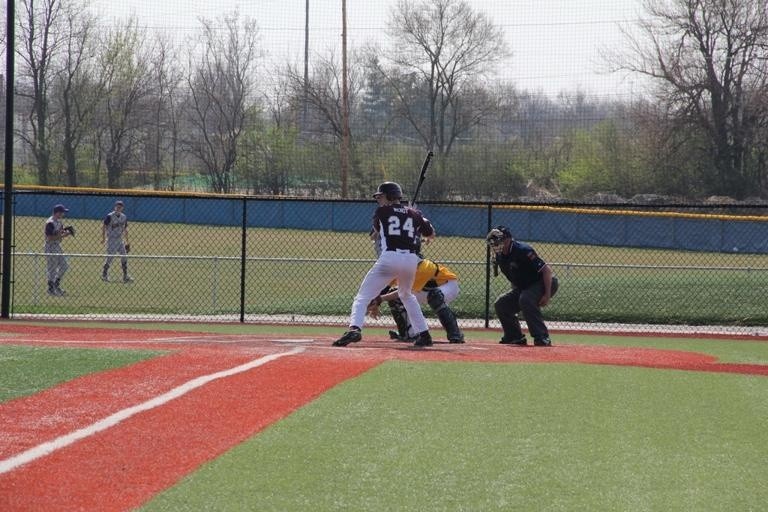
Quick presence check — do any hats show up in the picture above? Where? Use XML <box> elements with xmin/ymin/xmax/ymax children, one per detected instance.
<box><xmin>116</xmin><ymin>200</ymin><xmax>123</xmax><ymax>205</ymax></box>
<box><xmin>54</xmin><ymin>204</ymin><xmax>69</xmax><ymax>212</ymax></box>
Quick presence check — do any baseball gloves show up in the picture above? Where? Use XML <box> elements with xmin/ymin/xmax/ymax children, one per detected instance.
<box><xmin>124</xmin><ymin>243</ymin><xmax>130</xmax><ymax>253</ymax></box>
<box><xmin>365</xmin><ymin>296</ymin><xmax>382</xmax><ymax>320</ymax></box>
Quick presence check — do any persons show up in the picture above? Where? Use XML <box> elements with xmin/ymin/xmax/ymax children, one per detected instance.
<box><xmin>332</xmin><ymin>181</ymin><xmax>434</xmax><ymax>346</ymax></box>
<box><xmin>98</xmin><ymin>201</ymin><xmax>134</xmax><ymax>282</ymax></box>
<box><xmin>486</xmin><ymin>226</ymin><xmax>558</xmax><ymax>347</ymax></box>
<box><xmin>367</xmin><ymin>254</ymin><xmax>465</xmax><ymax>344</ymax></box>
<box><xmin>45</xmin><ymin>204</ymin><xmax>75</xmax><ymax>295</ymax></box>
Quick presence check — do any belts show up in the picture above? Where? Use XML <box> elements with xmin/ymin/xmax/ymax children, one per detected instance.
<box><xmin>387</xmin><ymin>247</ymin><xmax>414</xmax><ymax>253</ymax></box>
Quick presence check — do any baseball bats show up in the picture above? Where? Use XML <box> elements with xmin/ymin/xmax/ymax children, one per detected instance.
<box><xmin>411</xmin><ymin>152</ymin><xmax>434</xmax><ymax>207</ymax></box>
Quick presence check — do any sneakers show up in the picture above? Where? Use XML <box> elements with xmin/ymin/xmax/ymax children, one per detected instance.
<box><xmin>48</xmin><ymin>276</ymin><xmax>133</xmax><ymax>296</ymax></box>
<box><xmin>332</xmin><ymin>329</ymin><xmax>551</xmax><ymax>346</ymax></box>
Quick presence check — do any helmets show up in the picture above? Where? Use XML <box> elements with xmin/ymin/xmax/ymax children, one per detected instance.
<box><xmin>485</xmin><ymin>225</ymin><xmax>511</xmax><ymax>256</ymax></box>
<box><xmin>373</xmin><ymin>182</ymin><xmax>402</xmax><ymax>201</ymax></box>
